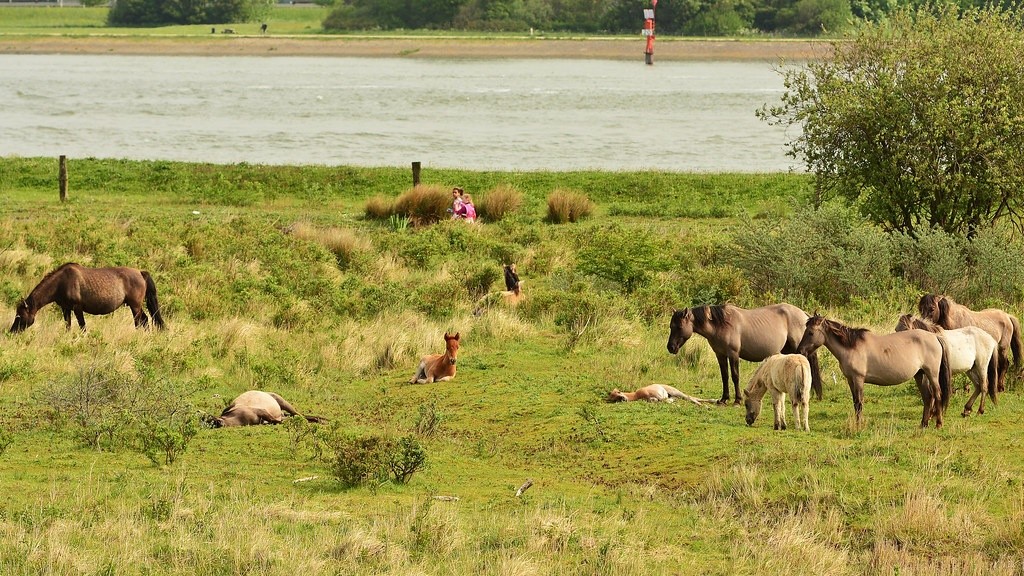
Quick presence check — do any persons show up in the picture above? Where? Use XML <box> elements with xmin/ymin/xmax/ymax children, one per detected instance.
<box><xmin>447</xmin><ymin>188</ymin><xmax>476</xmax><ymax>224</ymax></box>
<box><xmin>505</xmin><ymin>261</ymin><xmax>524</xmax><ymax>295</ymax></box>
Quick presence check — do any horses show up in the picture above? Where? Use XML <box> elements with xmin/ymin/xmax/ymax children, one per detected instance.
<box><xmin>405</xmin><ymin>332</ymin><xmax>461</xmax><ymax>386</ymax></box>
<box><xmin>201</xmin><ymin>389</ymin><xmax>326</xmax><ymax>428</ymax></box>
<box><xmin>9</xmin><ymin>262</ymin><xmax>164</xmax><ymax>334</ymax></box>
<box><xmin>797</xmin><ymin>292</ymin><xmax>1023</xmax><ymax>430</ymax></box>
<box><xmin>604</xmin><ymin>383</ymin><xmax>717</xmax><ymax>407</ymax></box>
<box><xmin>667</xmin><ymin>302</ymin><xmax>823</xmax><ymax>431</ymax></box>
